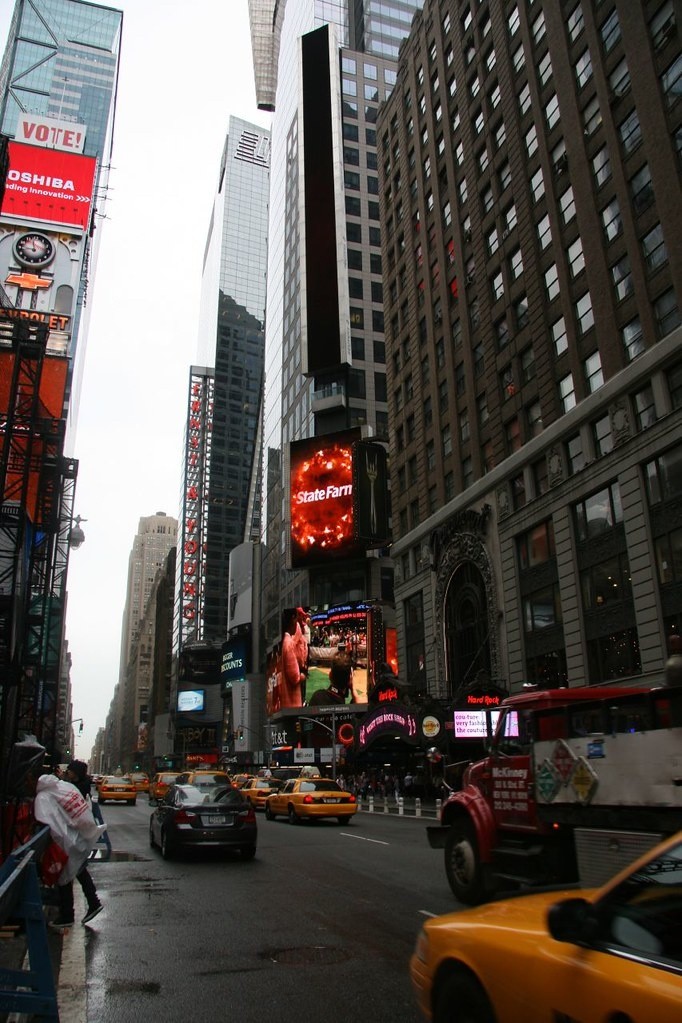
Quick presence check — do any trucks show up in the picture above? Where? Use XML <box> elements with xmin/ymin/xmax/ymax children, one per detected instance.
<box><xmin>425</xmin><ymin>649</ymin><xmax>682</xmax><ymax>906</ymax></box>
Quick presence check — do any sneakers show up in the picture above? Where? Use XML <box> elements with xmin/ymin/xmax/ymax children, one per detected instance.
<box><xmin>82</xmin><ymin>900</ymin><xmax>103</xmax><ymax>923</ymax></box>
<box><xmin>48</xmin><ymin>916</ymin><xmax>74</xmax><ymax>927</ymax></box>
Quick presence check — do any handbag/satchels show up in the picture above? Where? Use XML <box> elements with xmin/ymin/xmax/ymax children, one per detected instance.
<box><xmin>42</xmin><ymin>841</ymin><xmax>67</xmax><ymax>886</ymax></box>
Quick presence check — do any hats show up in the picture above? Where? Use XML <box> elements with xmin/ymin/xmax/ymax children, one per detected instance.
<box><xmin>67</xmin><ymin>761</ymin><xmax>87</xmax><ymax>775</ymax></box>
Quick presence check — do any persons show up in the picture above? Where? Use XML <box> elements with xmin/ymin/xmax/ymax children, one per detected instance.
<box><xmin>0</xmin><ymin>761</ymin><xmax>102</xmax><ymax>927</ymax></box>
<box><xmin>267</xmin><ymin>608</ymin><xmax>366</xmax><ymax>714</ymax></box>
<box><xmin>336</xmin><ymin>769</ymin><xmax>413</xmax><ymax>799</ymax></box>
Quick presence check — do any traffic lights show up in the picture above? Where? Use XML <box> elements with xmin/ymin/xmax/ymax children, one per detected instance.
<box><xmin>238</xmin><ymin>729</ymin><xmax>243</xmax><ymax>741</ymax></box>
<box><xmin>80</xmin><ymin>723</ymin><xmax>83</xmax><ymax>732</ymax></box>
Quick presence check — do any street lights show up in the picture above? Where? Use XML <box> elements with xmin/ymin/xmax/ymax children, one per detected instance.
<box><xmin>298</xmin><ymin>711</ymin><xmax>336</xmax><ymax>781</ymax></box>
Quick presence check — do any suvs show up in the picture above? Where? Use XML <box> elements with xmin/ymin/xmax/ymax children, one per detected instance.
<box><xmin>175</xmin><ymin>769</ymin><xmax>231</xmax><ymax>784</ymax></box>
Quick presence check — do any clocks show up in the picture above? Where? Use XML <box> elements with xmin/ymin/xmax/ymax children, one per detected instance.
<box><xmin>12</xmin><ymin>231</ymin><xmax>57</xmax><ymax>269</ymax></box>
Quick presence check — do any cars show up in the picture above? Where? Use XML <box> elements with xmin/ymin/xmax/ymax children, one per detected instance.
<box><xmin>236</xmin><ymin>777</ymin><xmax>283</xmax><ymax>813</ymax></box>
<box><xmin>148</xmin><ymin>772</ymin><xmax>180</xmax><ymax>806</ymax></box>
<box><xmin>410</xmin><ymin>830</ymin><xmax>681</xmax><ymax>1023</ymax></box>
<box><xmin>148</xmin><ymin>783</ymin><xmax>258</xmax><ymax>860</ymax></box>
<box><xmin>265</xmin><ymin>777</ymin><xmax>359</xmax><ymax>826</ymax></box>
<box><xmin>130</xmin><ymin>772</ymin><xmax>150</xmax><ymax>794</ymax></box>
<box><xmin>232</xmin><ymin>774</ymin><xmax>257</xmax><ymax>791</ymax></box>
<box><xmin>88</xmin><ymin>773</ymin><xmax>131</xmax><ymax>784</ymax></box>
<box><xmin>98</xmin><ymin>776</ymin><xmax>137</xmax><ymax>806</ymax></box>
